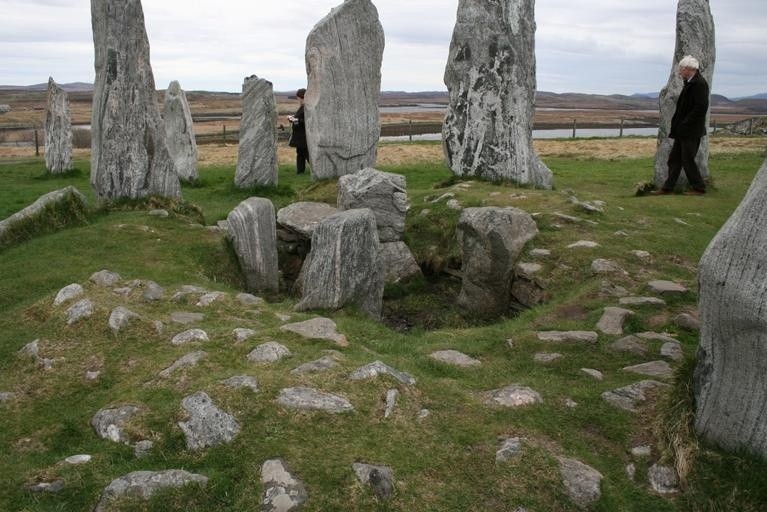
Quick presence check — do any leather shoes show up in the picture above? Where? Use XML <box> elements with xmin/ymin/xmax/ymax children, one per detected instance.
<box><xmin>649</xmin><ymin>189</ymin><xmax>673</xmax><ymax>195</ymax></box>
<box><xmin>682</xmin><ymin>190</ymin><xmax>703</xmax><ymax>195</ymax></box>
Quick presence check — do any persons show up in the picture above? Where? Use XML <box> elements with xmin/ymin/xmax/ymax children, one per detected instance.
<box><xmin>286</xmin><ymin>87</ymin><xmax>309</xmax><ymax>175</ymax></box>
<box><xmin>649</xmin><ymin>54</ymin><xmax>710</xmax><ymax>196</ymax></box>
